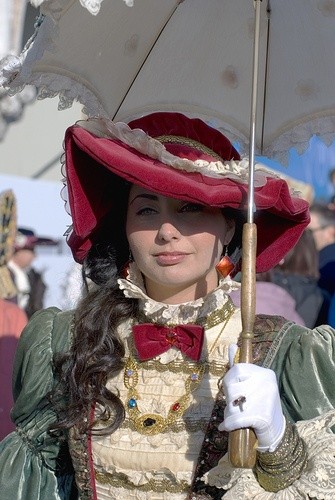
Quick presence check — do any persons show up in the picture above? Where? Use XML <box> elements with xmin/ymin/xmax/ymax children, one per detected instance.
<box><xmin>0</xmin><ymin>188</ymin><xmax>28</xmax><ymax>442</ymax></box>
<box><xmin>3</xmin><ymin>227</ymin><xmax>48</xmax><ymax>321</ymax></box>
<box><xmin>0</xmin><ymin>111</ymin><xmax>335</xmax><ymax>500</ymax></box>
<box><xmin>229</xmin><ymin>204</ymin><xmax>335</xmax><ymax>330</ymax></box>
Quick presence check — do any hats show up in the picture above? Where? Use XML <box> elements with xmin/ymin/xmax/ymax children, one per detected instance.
<box><xmin>60</xmin><ymin>108</ymin><xmax>312</xmax><ymax>274</ymax></box>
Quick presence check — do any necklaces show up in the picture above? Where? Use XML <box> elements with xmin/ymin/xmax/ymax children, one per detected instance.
<box><xmin>124</xmin><ymin>293</ymin><xmax>237</xmax><ymax>436</ymax></box>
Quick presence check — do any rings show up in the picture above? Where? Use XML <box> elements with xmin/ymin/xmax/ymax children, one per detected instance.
<box><xmin>232</xmin><ymin>396</ymin><xmax>246</xmax><ymax>412</ymax></box>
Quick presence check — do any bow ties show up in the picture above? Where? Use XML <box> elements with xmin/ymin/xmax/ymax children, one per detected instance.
<box><xmin>132</xmin><ymin>324</ymin><xmax>204</xmax><ymax>361</ymax></box>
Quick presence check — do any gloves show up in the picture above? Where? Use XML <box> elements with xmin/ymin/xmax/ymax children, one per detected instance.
<box><xmin>218</xmin><ymin>343</ymin><xmax>288</xmax><ymax>452</ymax></box>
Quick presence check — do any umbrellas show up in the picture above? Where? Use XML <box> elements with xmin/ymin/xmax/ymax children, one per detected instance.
<box><xmin>0</xmin><ymin>0</ymin><xmax>335</xmax><ymax>468</ymax></box>
